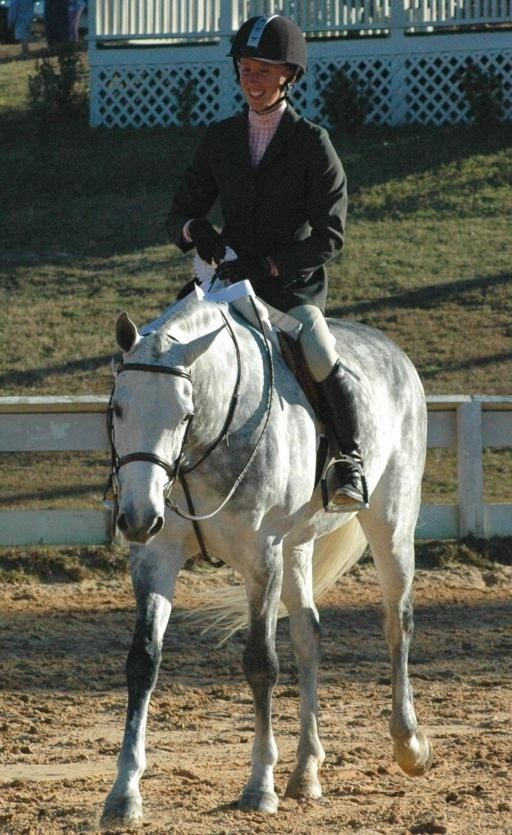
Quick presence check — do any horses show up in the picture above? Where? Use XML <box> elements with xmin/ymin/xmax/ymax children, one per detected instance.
<box><xmin>98</xmin><ymin>296</ymin><xmax>435</xmax><ymax>831</ymax></box>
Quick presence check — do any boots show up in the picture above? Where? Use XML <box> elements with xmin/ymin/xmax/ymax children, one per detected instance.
<box><xmin>317</xmin><ymin>360</ymin><xmax>363</xmax><ymax>505</ymax></box>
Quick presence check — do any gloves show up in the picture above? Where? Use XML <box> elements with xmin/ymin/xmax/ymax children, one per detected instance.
<box><xmin>189</xmin><ymin>218</ymin><xmax>225</xmax><ymax>265</ymax></box>
<box><xmin>215</xmin><ymin>249</ymin><xmax>265</xmax><ymax>282</ymax></box>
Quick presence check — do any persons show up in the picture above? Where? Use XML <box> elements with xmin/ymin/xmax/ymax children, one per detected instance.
<box><xmin>69</xmin><ymin>1</ymin><xmax>86</xmax><ymax>43</ymax></box>
<box><xmin>7</xmin><ymin>1</ymin><xmax>36</xmax><ymax>52</ymax></box>
<box><xmin>164</xmin><ymin>15</ymin><xmax>366</xmax><ymax>506</ymax></box>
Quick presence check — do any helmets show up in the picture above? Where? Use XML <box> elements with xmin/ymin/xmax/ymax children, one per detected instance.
<box><xmin>227</xmin><ymin>14</ymin><xmax>307</xmax><ymax>75</ymax></box>
